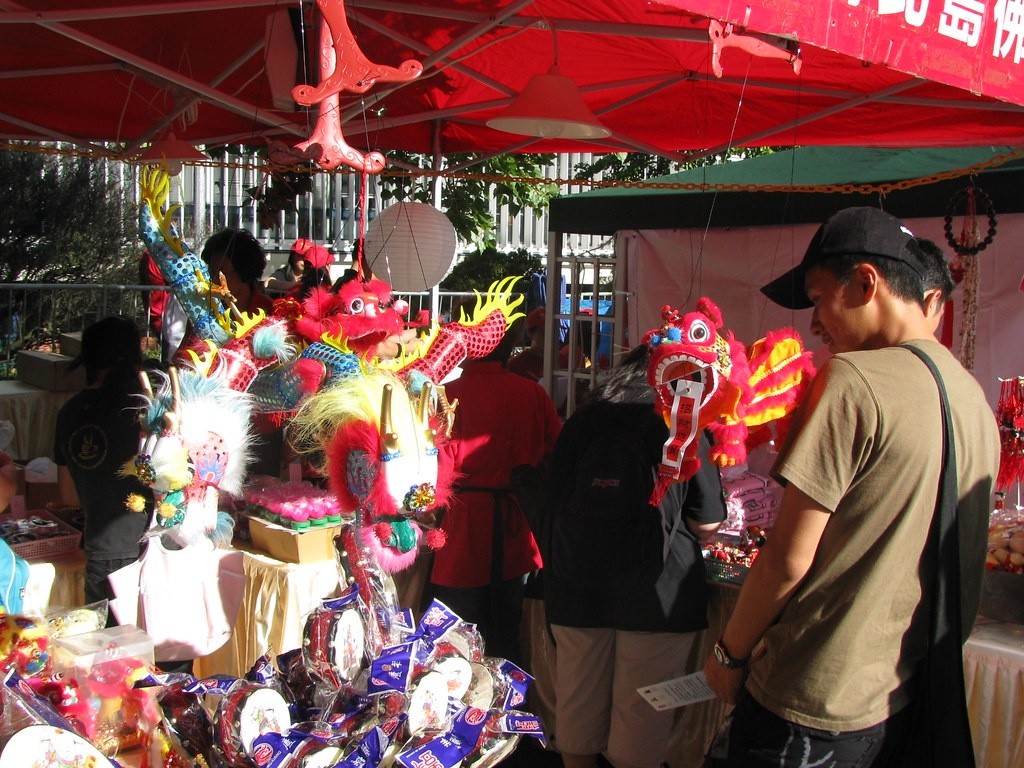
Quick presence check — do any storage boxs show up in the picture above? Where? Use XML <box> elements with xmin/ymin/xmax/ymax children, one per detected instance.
<box><xmin>247</xmin><ymin>519</ymin><xmax>342</xmax><ymax>563</ymax></box>
<box><xmin>701</xmin><ymin>557</ymin><xmax>748</xmax><ymax>585</ymax></box>
<box><xmin>52</xmin><ymin>624</ymin><xmax>156</xmax><ymax>708</ymax></box>
<box><xmin>0</xmin><ymin>506</ymin><xmax>82</xmax><ymax>559</ymax></box>
<box><xmin>59</xmin><ymin>331</ymin><xmax>81</xmax><ymax>357</ymax></box>
<box><xmin>15</xmin><ymin>349</ymin><xmax>87</xmax><ymax>394</ymax></box>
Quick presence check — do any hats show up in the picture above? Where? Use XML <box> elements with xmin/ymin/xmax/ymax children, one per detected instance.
<box><xmin>291</xmin><ymin>238</ymin><xmax>314</xmax><ymax>255</ymax></box>
<box><xmin>582</xmin><ymin>309</ymin><xmax>595</xmax><ymax>316</ymax></box>
<box><xmin>759</xmin><ymin>205</ymin><xmax>928</xmax><ymax>313</ymax></box>
<box><xmin>304</xmin><ymin>246</ymin><xmax>336</xmax><ymax>270</ymax></box>
<box><xmin>71</xmin><ymin>317</ymin><xmax>138</xmax><ymax>368</ymax></box>
<box><xmin>525</xmin><ymin>307</ymin><xmax>545</xmax><ymax>330</ymax></box>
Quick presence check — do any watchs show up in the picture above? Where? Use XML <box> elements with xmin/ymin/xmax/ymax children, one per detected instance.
<box><xmin>715</xmin><ymin>635</ymin><xmax>747</xmax><ymax>670</ymax></box>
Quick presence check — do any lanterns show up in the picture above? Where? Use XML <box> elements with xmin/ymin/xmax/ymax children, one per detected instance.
<box><xmin>363</xmin><ymin>201</ymin><xmax>457</xmax><ymax>292</ymax></box>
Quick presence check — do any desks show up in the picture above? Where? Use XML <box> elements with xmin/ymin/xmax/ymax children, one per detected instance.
<box><xmin>0</xmin><ymin>379</ymin><xmax>80</xmax><ymax>461</ymax></box>
<box><xmin>31</xmin><ymin>550</ymin><xmax>88</xmax><ymax>609</ymax></box>
<box><xmin>193</xmin><ymin>541</ymin><xmax>434</xmax><ymax>684</ymax></box>
<box><xmin>522</xmin><ymin>553</ymin><xmax>1024</xmax><ymax>768</ymax></box>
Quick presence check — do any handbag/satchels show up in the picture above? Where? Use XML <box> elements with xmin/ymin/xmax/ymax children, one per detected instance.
<box><xmin>905</xmin><ymin>633</ymin><xmax>975</xmax><ymax>768</ymax></box>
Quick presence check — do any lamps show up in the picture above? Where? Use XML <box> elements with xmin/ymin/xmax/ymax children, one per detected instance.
<box><xmin>139</xmin><ymin>129</ymin><xmax>209</xmax><ymax>177</ymax></box>
<box><xmin>486</xmin><ymin>21</ymin><xmax>613</xmax><ymax>139</ymax></box>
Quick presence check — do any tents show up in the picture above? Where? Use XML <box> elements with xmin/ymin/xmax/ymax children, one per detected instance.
<box><xmin>543</xmin><ymin>144</ymin><xmax>1024</xmax><ymax>424</ymax></box>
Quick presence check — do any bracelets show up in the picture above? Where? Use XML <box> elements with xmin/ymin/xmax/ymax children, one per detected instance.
<box><xmin>944</xmin><ymin>188</ymin><xmax>997</xmax><ymax>256</ymax></box>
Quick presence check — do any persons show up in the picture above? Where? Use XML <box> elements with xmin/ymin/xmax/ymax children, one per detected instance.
<box><xmin>701</xmin><ymin>208</ymin><xmax>1000</xmax><ymax>768</ymax></box>
<box><xmin>423</xmin><ymin>298</ymin><xmax>560</xmax><ymax>668</ymax></box>
<box><xmin>141</xmin><ymin>229</ymin><xmax>381</xmax><ymax>491</ymax></box>
<box><xmin>534</xmin><ymin>344</ymin><xmax>713</xmax><ymax>768</ymax></box>
<box><xmin>52</xmin><ymin>315</ymin><xmax>154</xmax><ymax>626</ymax></box>
<box><xmin>510</xmin><ymin>301</ymin><xmax>615</xmax><ymax>382</ymax></box>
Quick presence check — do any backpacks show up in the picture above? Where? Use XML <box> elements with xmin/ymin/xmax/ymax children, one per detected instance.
<box><xmin>552</xmin><ymin>404</ymin><xmax>684</xmax><ymax>590</ymax></box>
